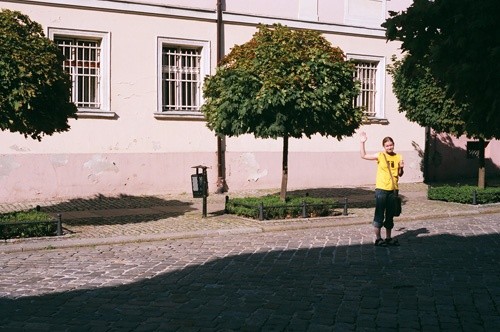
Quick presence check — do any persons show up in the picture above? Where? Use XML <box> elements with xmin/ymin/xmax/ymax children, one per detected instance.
<box><xmin>359</xmin><ymin>131</ymin><xmax>405</xmax><ymax>248</ymax></box>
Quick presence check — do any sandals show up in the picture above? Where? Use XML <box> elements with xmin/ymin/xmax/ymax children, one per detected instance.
<box><xmin>374</xmin><ymin>238</ymin><xmax>389</xmax><ymax>247</ymax></box>
<box><xmin>385</xmin><ymin>238</ymin><xmax>400</xmax><ymax>246</ymax></box>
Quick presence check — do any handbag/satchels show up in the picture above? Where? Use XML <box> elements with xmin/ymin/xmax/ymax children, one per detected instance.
<box><xmin>393</xmin><ymin>196</ymin><xmax>402</xmax><ymax>217</ymax></box>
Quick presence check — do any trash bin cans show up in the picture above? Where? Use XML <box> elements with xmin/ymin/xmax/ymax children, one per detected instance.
<box><xmin>191</xmin><ymin>165</ymin><xmax>209</xmax><ymax>217</ymax></box>
